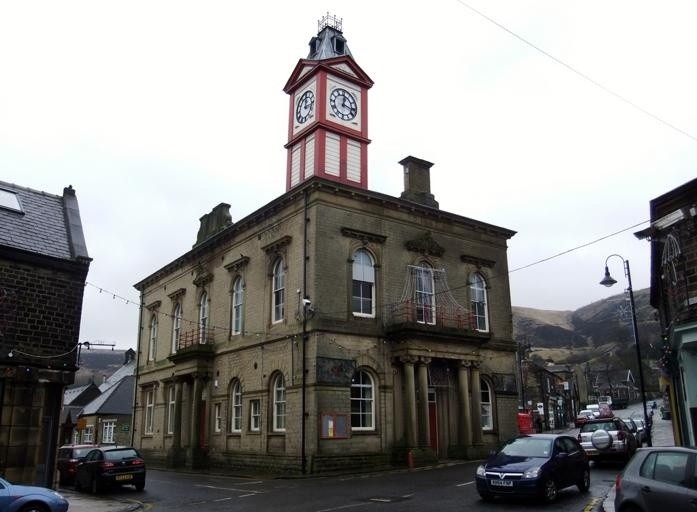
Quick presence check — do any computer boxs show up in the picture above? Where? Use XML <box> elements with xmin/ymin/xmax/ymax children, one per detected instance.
<box><xmin>301</xmin><ymin>298</ymin><xmax>312</xmax><ymax>309</ymax></box>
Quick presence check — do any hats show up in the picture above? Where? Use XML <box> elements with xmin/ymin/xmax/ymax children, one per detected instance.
<box><xmin>0</xmin><ymin>478</ymin><xmax>68</xmax><ymax>512</ymax></box>
<box><xmin>605</xmin><ymin>419</ymin><xmax>646</xmax><ymax>445</ymax></box>
<box><xmin>475</xmin><ymin>432</ymin><xmax>591</xmax><ymax>501</ymax></box>
<box><xmin>614</xmin><ymin>445</ymin><xmax>697</xmax><ymax>512</ymax></box>
<box><xmin>577</xmin><ymin>394</ymin><xmax>613</xmax><ymax>424</ymax></box>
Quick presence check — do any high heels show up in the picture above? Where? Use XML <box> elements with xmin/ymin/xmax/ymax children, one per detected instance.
<box><xmin>406</xmin><ymin>451</ymin><xmax>414</xmax><ymax>468</ymax></box>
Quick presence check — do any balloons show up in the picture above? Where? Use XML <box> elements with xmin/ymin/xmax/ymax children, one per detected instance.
<box><xmin>293</xmin><ymin>80</ymin><xmax>316</xmax><ymax>136</ymax></box>
<box><xmin>326</xmin><ymin>78</ymin><xmax>361</xmax><ymax>132</ymax></box>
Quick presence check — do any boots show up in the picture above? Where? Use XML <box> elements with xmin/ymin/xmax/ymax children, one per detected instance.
<box><xmin>579</xmin><ymin>417</ymin><xmax>637</xmax><ymax>463</ymax></box>
<box><xmin>57</xmin><ymin>443</ymin><xmax>99</xmax><ymax>484</ymax></box>
<box><xmin>73</xmin><ymin>445</ymin><xmax>146</xmax><ymax>494</ymax></box>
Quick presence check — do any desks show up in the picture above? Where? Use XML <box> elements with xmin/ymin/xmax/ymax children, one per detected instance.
<box><xmin>602</xmin><ymin>251</ymin><xmax>653</xmax><ymax>447</ymax></box>
